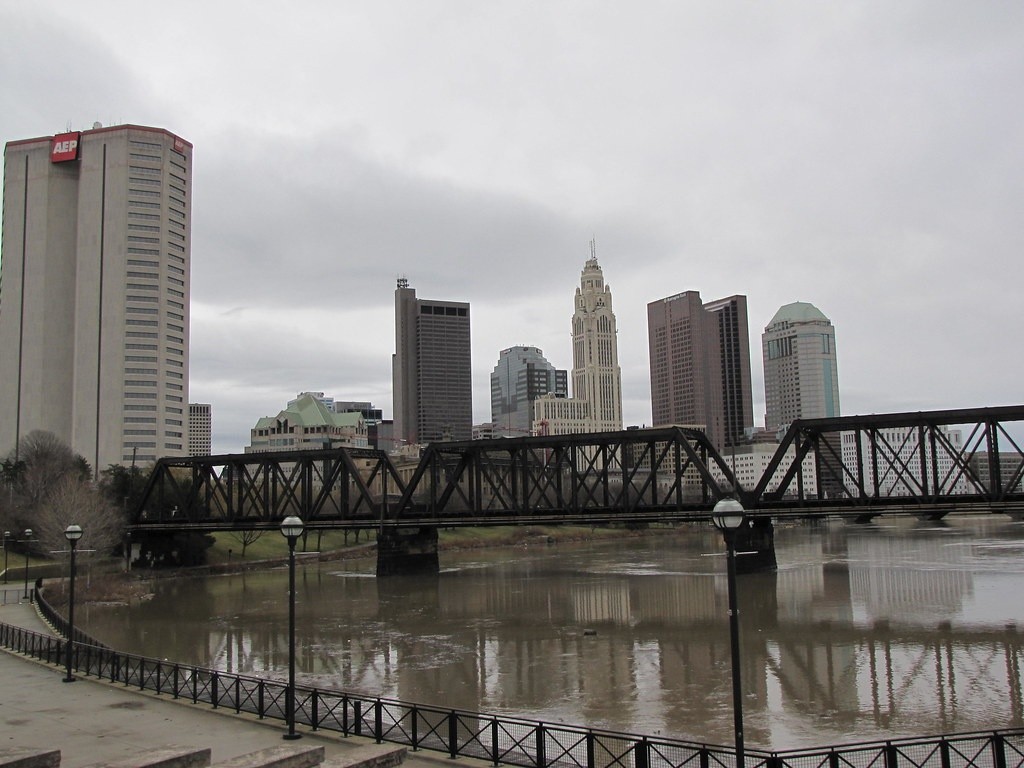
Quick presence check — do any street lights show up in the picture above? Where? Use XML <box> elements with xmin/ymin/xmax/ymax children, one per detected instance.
<box><xmin>280</xmin><ymin>516</ymin><xmax>305</xmax><ymax>740</ymax></box>
<box><xmin>4</xmin><ymin>531</ymin><xmax>10</xmax><ymax>584</ymax></box>
<box><xmin>23</xmin><ymin>529</ymin><xmax>32</xmax><ymax>599</ymax></box>
<box><xmin>711</xmin><ymin>496</ymin><xmax>746</xmax><ymax>768</ymax></box>
<box><xmin>62</xmin><ymin>525</ymin><xmax>83</xmax><ymax>682</ymax></box>
<box><xmin>127</xmin><ymin>532</ymin><xmax>131</xmax><ymax>572</ymax></box>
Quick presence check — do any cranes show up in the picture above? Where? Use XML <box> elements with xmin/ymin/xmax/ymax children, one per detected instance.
<box><xmin>472</xmin><ymin>421</ymin><xmax>550</xmax><ymax>473</ymax></box>
<box><xmin>335</xmin><ymin>432</ymin><xmax>414</xmax><ymax>445</ymax></box>
